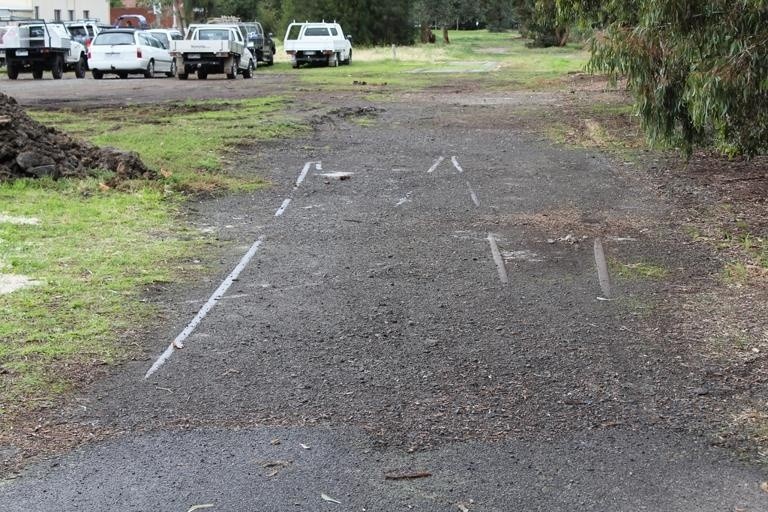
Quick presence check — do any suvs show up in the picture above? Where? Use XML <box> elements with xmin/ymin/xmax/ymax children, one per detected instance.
<box><xmin>0</xmin><ymin>13</ymin><xmax>275</xmax><ymax>81</ymax></box>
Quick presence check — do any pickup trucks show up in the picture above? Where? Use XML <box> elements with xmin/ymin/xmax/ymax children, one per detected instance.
<box><xmin>284</xmin><ymin>20</ymin><xmax>353</xmax><ymax>67</ymax></box>
